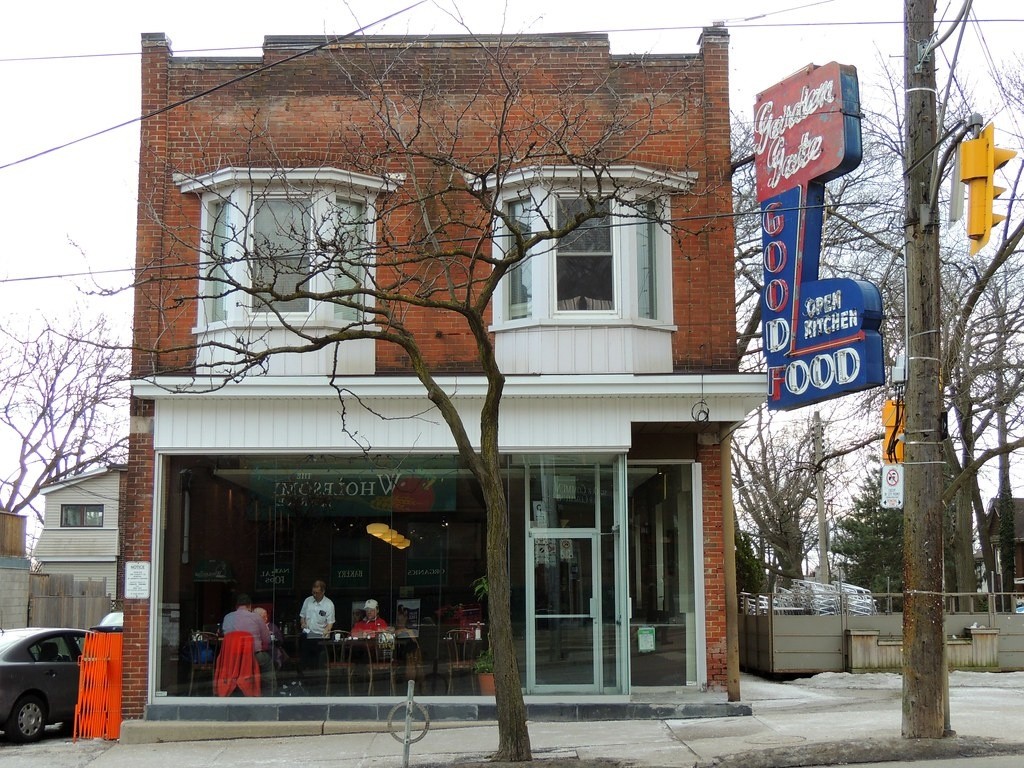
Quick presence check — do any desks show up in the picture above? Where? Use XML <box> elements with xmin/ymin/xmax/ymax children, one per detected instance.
<box><xmin>318</xmin><ymin>637</ymin><xmax>408</xmax><ymax>644</ymax></box>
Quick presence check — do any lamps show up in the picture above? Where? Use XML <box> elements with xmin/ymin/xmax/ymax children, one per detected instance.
<box><xmin>367</xmin><ymin>523</ymin><xmax>410</xmax><ymax>549</ymax></box>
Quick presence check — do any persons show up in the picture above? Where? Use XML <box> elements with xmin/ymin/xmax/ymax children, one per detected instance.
<box><xmin>350</xmin><ymin>600</ymin><xmax>388</xmax><ymax>636</ymax></box>
<box><xmin>646</xmin><ymin>583</ymin><xmax>673</xmax><ymax>644</ymax></box>
<box><xmin>300</xmin><ymin>580</ymin><xmax>335</xmax><ymax>634</ymax></box>
<box><xmin>222</xmin><ymin>593</ymin><xmax>283</xmax><ymax>697</ymax></box>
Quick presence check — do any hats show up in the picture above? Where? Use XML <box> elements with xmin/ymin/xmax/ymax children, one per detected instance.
<box><xmin>363</xmin><ymin>599</ymin><xmax>377</xmax><ymax>609</ymax></box>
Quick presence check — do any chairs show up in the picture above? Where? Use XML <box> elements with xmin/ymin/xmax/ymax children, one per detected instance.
<box><xmin>188</xmin><ymin>631</ymin><xmax>219</xmax><ymax>697</ymax></box>
<box><xmin>445</xmin><ymin>630</ymin><xmax>478</xmax><ymax>695</ymax></box>
<box><xmin>364</xmin><ymin>631</ymin><xmax>398</xmax><ymax>697</ymax></box>
<box><xmin>37</xmin><ymin>642</ymin><xmax>62</xmax><ymax>662</ymax></box>
<box><xmin>324</xmin><ymin>630</ymin><xmax>355</xmax><ymax>696</ymax></box>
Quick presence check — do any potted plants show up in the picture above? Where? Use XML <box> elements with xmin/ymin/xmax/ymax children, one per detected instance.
<box><xmin>469</xmin><ymin>575</ymin><xmax>495</xmax><ymax>695</ymax></box>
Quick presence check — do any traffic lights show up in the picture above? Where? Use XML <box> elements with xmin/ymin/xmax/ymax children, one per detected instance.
<box><xmin>959</xmin><ymin>123</ymin><xmax>1018</xmax><ymax>256</ymax></box>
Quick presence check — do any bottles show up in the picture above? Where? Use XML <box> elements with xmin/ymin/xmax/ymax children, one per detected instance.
<box><xmin>216</xmin><ymin>623</ymin><xmax>220</xmax><ymax>639</ymax></box>
<box><xmin>283</xmin><ymin>625</ymin><xmax>288</xmax><ymax>634</ymax></box>
<box><xmin>375</xmin><ymin>623</ymin><xmax>382</xmax><ymax>643</ymax></box>
<box><xmin>279</xmin><ymin>622</ymin><xmax>283</xmax><ymax>631</ymax></box>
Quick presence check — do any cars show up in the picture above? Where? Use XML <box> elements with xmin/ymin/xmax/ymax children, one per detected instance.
<box><xmin>90</xmin><ymin>612</ymin><xmax>123</xmax><ymax>633</ymax></box>
<box><xmin>0</xmin><ymin>627</ymin><xmax>96</xmax><ymax>743</ymax></box>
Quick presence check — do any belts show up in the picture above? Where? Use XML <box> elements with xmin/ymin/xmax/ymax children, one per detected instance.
<box><xmin>255</xmin><ymin>650</ymin><xmax>264</xmax><ymax>654</ymax></box>
